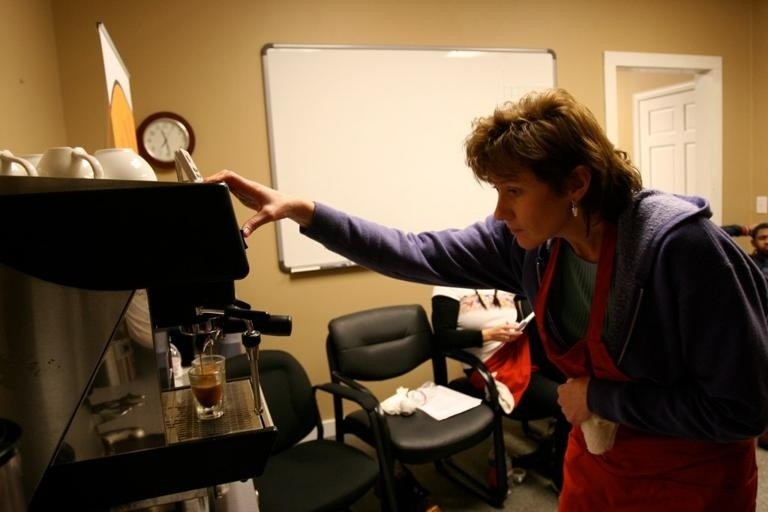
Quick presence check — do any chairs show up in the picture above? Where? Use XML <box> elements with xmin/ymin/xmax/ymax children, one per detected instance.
<box><xmin>434</xmin><ymin>346</ymin><xmax>561</xmax><ymax>508</ymax></box>
<box><xmin>326</xmin><ymin>304</ymin><xmax>508</xmax><ymax>512</ymax></box>
<box><xmin>225</xmin><ymin>350</ymin><xmax>398</xmax><ymax>512</ymax></box>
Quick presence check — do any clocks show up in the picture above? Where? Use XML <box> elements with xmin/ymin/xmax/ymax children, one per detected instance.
<box><xmin>136</xmin><ymin>112</ymin><xmax>195</xmax><ymax>169</ymax></box>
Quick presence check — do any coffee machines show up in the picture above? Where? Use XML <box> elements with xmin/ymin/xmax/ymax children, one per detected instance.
<box><xmin>0</xmin><ymin>176</ymin><xmax>292</xmax><ymax>512</ymax></box>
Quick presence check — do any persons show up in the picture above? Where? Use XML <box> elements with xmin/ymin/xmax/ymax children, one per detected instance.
<box><xmin>192</xmin><ymin>88</ymin><xmax>768</xmax><ymax>512</ymax></box>
<box><xmin>432</xmin><ymin>284</ymin><xmax>567</xmax><ymax>495</ymax></box>
<box><xmin>720</xmin><ymin>224</ymin><xmax>767</xmax><ymax>454</ymax></box>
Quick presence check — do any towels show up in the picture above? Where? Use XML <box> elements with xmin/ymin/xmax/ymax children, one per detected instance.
<box><xmin>580</xmin><ymin>416</ymin><xmax>618</xmax><ymax>454</ymax></box>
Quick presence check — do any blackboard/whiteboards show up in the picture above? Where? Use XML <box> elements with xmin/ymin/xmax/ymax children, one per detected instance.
<box><xmin>261</xmin><ymin>41</ymin><xmax>559</xmax><ymax>275</ymax></box>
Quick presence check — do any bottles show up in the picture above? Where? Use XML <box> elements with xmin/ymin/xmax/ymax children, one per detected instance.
<box><xmin>504</xmin><ymin>451</ymin><xmax>513</xmax><ymax>496</ymax></box>
<box><xmin>488</xmin><ymin>444</ymin><xmax>497</xmax><ymax>489</ymax></box>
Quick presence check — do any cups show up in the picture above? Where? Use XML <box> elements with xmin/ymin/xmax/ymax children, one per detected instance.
<box><xmin>188</xmin><ymin>364</ymin><xmax>222</xmax><ymax>421</ymax></box>
<box><xmin>191</xmin><ymin>355</ymin><xmax>227</xmax><ymax>413</ymax></box>
<box><xmin>513</xmin><ymin>467</ymin><xmax>526</xmax><ymax>485</ymax></box>
<box><xmin>0</xmin><ymin>147</ymin><xmax>158</xmax><ymax>181</ymax></box>
